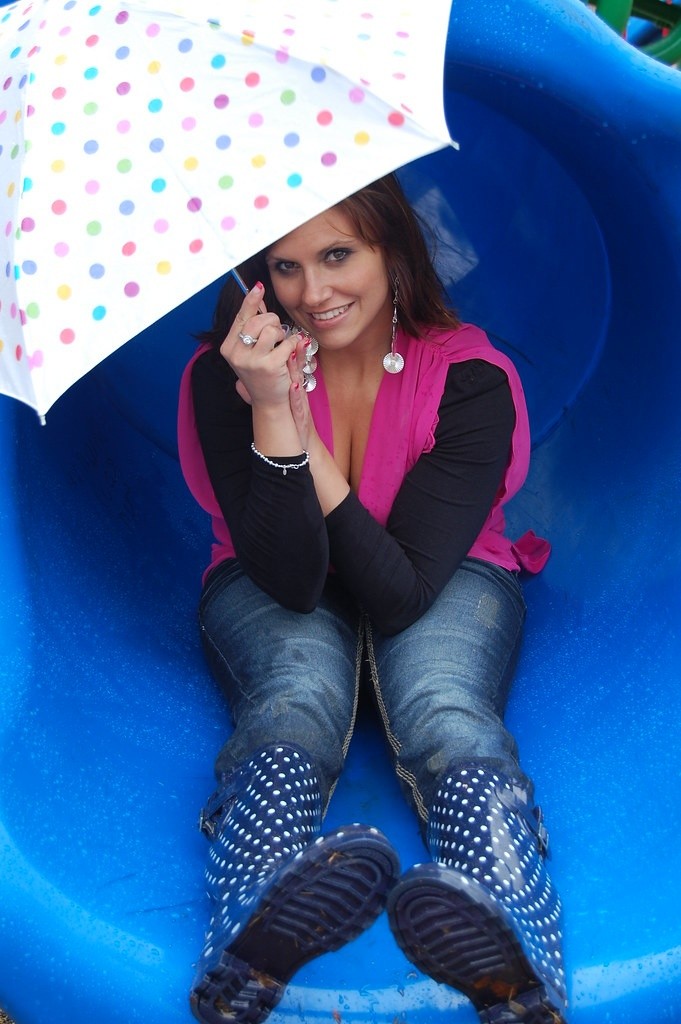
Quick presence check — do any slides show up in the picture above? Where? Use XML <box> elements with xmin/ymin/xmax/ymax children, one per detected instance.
<box><xmin>1</xmin><ymin>0</ymin><xmax>681</xmax><ymax>1024</ymax></box>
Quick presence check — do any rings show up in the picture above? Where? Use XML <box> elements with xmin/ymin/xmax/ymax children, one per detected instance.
<box><xmin>240</xmin><ymin>332</ymin><xmax>258</xmax><ymax>347</ymax></box>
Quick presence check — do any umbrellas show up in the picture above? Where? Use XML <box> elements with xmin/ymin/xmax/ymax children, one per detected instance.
<box><xmin>0</xmin><ymin>0</ymin><xmax>460</xmax><ymax>426</ymax></box>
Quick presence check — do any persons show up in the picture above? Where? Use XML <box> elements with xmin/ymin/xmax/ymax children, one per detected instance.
<box><xmin>176</xmin><ymin>172</ymin><xmax>573</xmax><ymax>1024</ymax></box>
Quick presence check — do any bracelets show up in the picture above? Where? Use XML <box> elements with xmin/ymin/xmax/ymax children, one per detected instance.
<box><xmin>250</xmin><ymin>443</ymin><xmax>312</xmax><ymax>470</ymax></box>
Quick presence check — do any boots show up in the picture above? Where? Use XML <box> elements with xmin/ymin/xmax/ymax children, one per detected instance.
<box><xmin>191</xmin><ymin>740</ymin><xmax>400</xmax><ymax>1024</ymax></box>
<box><xmin>387</xmin><ymin>764</ymin><xmax>569</xmax><ymax>1024</ymax></box>
